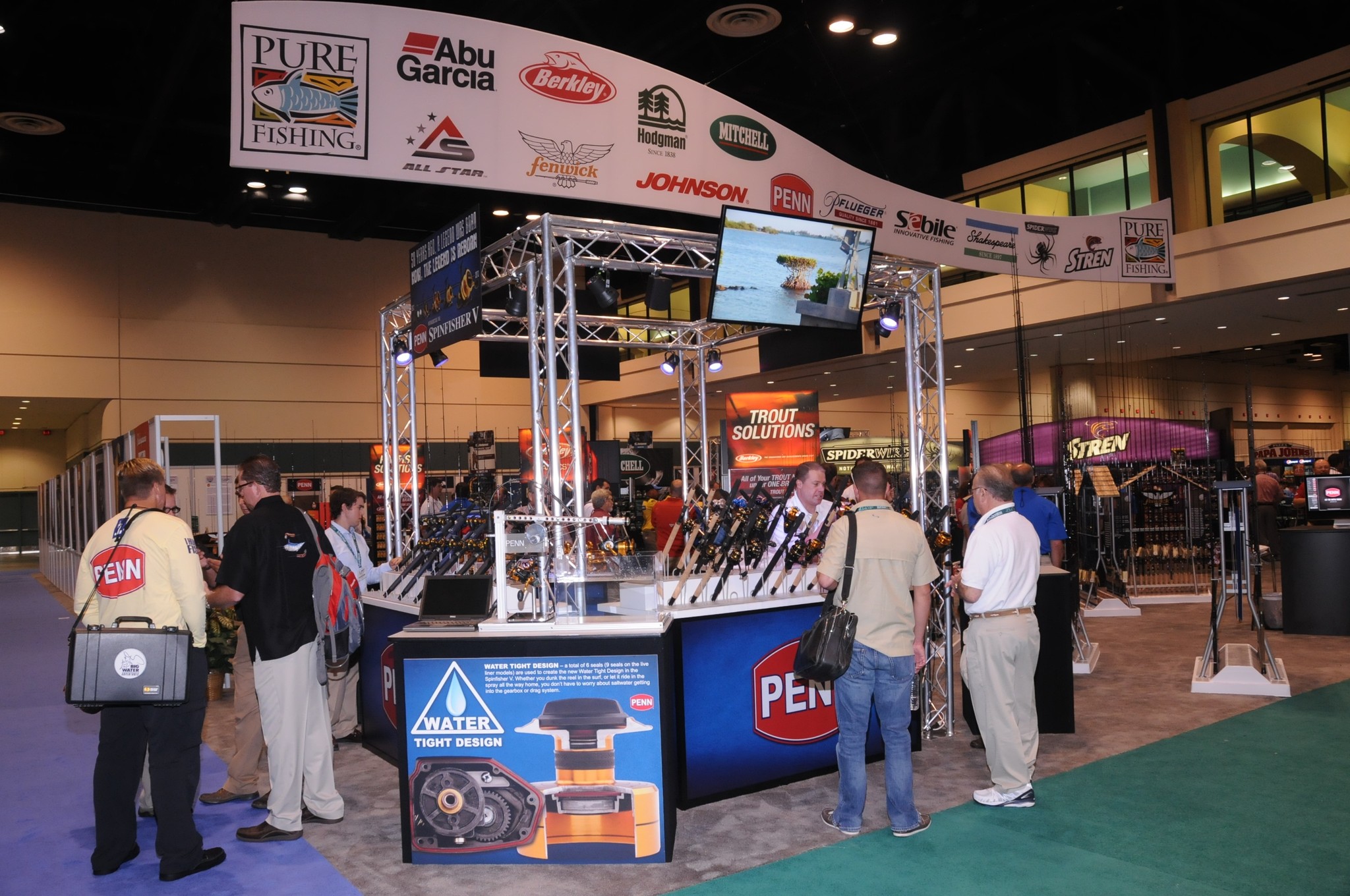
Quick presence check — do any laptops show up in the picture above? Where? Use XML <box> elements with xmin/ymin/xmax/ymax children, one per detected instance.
<box><xmin>403</xmin><ymin>575</ymin><xmax>492</xmax><ymax>632</ymax></box>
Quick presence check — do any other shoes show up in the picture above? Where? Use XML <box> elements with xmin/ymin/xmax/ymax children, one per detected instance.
<box><xmin>138</xmin><ymin>806</ymin><xmax>155</xmax><ymax>817</ymax></box>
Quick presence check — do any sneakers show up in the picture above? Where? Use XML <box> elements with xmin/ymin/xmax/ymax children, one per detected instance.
<box><xmin>972</xmin><ymin>786</ymin><xmax>1035</xmax><ymax>807</ymax></box>
<box><xmin>822</xmin><ymin>807</ymin><xmax>859</xmax><ymax>835</ymax></box>
<box><xmin>893</xmin><ymin>814</ymin><xmax>932</xmax><ymax>837</ymax></box>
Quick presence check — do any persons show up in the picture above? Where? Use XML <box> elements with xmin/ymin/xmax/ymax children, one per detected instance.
<box><xmin>1293</xmin><ymin>454</ymin><xmax>1344</xmax><ymax>526</ymax></box>
<box><xmin>840</xmin><ymin>456</ymin><xmax>894</xmax><ymax>508</ymax></box>
<box><xmin>819</xmin><ymin>463</ymin><xmax>838</xmax><ymax>502</ymax></box>
<box><xmin>439</xmin><ymin>482</ymin><xmax>479</xmax><ymax>520</ymax></box>
<box><xmin>1246</xmin><ymin>459</ymin><xmax>1284</xmax><ymax>545</ymax></box>
<box><xmin>324</xmin><ymin>488</ymin><xmax>403</xmax><ymax>751</ymax></box>
<box><xmin>695</xmin><ymin>480</ymin><xmax>719</xmax><ymax>506</ymax></box>
<box><xmin>642</xmin><ymin>483</ymin><xmax>670</xmax><ymax>554</ymax></box>
<box><xmin>762</xmin><ymin>461</ymin><xmax>839</xmax><ymax>567</ymax></box>
<box><xmin>817</xmin><ymin>461</ymin><xmax>940</xmax><ymax>836</ymax></box>
<box><xmin>585</xmin><ymin>489</ymin><xmax>619</xmax><ymax>550</ymax></box>
<box><xmin>490</xmin><ymin>486</ymin><xmax>510</xmax><ymax>511</ymax></box>
<box><xmin>74</xmin><ymin>458</ymin><xmax>226</xmax><ymax>881</ymax></box>
<box><xmin>420</xmin><ymin>478</ymin><xmax>443</xmax><ymax>538</ymax></box>
<box><xmin>651</xmin><ymin>479</ymin><xmax>695</xmax><ymax>572</ymax></box>
<box><xmin>584</xmin><ymin>478</ymin><xmax>610</xmax><ymax>517</ymax></box>
<box><xmin>955</xmin><ymin>478</ymin><xmax>982</xmax><ymax>558</ymax></box>
<box><xmin>944</xmin><ymin>463</ymin><xmax>1041</xmax><ymax>807</ymax></box>
<box><xmin>1011</xmin><ymin>464</ymin><xmax>1068</xmax><ymax>568</ymax></box>
<box><xmin>204</xmin><ymin>454</ymin><xmax>344</xmax><ymax>840</ymax></box>
<box><xmin>505</xmin><ymin>477</ymin><xmax>573</xmax><ymax>544</ymax></box>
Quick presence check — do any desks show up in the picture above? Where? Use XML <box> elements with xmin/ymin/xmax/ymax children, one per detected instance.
<box><xmin>387</xmin><ymin>614</ymin><xmax>678</xmax><ymax>865</ymax></box>
<box><xmin>1278</xmin><ymin>526</ymin><xmax>1350</xmax><ymax>636</ymax></box>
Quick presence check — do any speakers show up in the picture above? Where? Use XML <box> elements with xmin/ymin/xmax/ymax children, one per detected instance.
<box><xmin>644</xmin><ymin>275</ymin><xmax>673</xmax><ymax>311</ymax></box>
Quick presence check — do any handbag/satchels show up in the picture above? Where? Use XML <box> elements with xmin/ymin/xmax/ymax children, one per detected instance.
<box><xmin>793</xmin><ymin>605</ymin><xmax>858</xmax><ymax>682</ymax></box>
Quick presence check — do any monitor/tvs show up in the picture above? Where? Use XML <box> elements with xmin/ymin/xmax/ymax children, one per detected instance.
<box><xmin>1304</xmin><ymin>474</ymin><xmax>1350</xmax><ymax>525</ymax></box>
<box><xmin>707</xmin><ymin>205</ymin><xmax>878</xmax><ymax>335</ymax></box>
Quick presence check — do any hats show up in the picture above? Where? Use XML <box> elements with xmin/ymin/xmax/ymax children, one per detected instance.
<box><xmin>645</xmin><ymin>484</ymin><xmax>662</xmax><ymax>493</ymax></box>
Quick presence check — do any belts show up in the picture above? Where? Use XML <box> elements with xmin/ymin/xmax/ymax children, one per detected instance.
<box><xmin>972</xmin><ymin>608</ymin><xmax>1034</xmax><ymax>620</ymax></box>
<box><xmin>1042</xmin><ymin>553</ymin><xmax>1049</xmax><ymax>555</ymax></box>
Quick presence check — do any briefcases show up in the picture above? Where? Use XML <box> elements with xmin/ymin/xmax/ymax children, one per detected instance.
<box><xmin>65</xmin><ymin>615</ymin><xmax>194</xmax><ymax>710</ymax></box>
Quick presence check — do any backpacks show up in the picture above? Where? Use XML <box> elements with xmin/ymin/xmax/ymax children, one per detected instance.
<box><xmin>295</xmin><ymin>506</ymin><xmax>364</xmax><ymax>684</ymax></box>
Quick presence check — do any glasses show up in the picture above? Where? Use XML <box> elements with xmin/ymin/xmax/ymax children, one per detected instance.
<box><xmin>163</xmin><ymin>506</ymin><xmax>180</xmax><ymax>513</ymax></box>
<box><xmin>235</xmin><ymin>481</ymin><xmax>266</xmax><ymax>492</ymax></box>
<box><xmin>851</xmin><ymin>468</ymin><xmax>858</xmax><ymax>493</ymax></box>
<box><xmin>968</xmin><ymin>488</ymin><xmax>987</xmax><ymax>494</ymax></box>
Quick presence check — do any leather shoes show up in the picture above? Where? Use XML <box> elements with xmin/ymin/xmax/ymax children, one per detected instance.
<box><xmin>336</xmin><ymin>728</ymin><xmax>363</xmax><ymax>742</ymax></box>
<box><xmin>331</xmin><ymin>736</ymin><xmax>339</xmax><ymax>750</ymax></box>
<box><xmin>236</xmin><ymin>820</ymin><xmax>303</xmax><ymax>841</ymax></box>
<box><xmin>970</xmin><ymin>738</ymin><xmax>986</xmax><ymax>749</ymax></box>
<box><xmin>93</xmin><ymin>842</ymin><xmax>140</xmax><ymax>876</ymax></box>
<box><xmin>199</xmin><ymin>788</ymin><xmax>260</xmax><ymax>803</ymax></box>
<box><xmin>251</xmin><ymin>791</ymin><xmax>271</xmax><ymax>808</ymax></box>
<box><xmin>160</xmin><ymin>847</ymin><xmax>226</xmax><ymax>881</ymax></box>
<box><xmin>302</xmin><ymin>806</ymin><xmax>344</xmax><ymax>824</ymax></box>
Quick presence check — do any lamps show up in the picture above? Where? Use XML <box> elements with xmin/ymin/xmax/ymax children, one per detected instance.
<box><xmin>587</xmin><ymin>267</ymin><xmax>619</xmax><ymax>307</ymax></box>
<box><xmin>392</xmin><ymin>334</ymin><xmax>413</xmax><ymax>366</ymax></box>
<box><xmin>840</xmin><ymin>230</ymin><xmax>857</xmax><ymax>254</ymax></box>
<box><xmin>661</xmin><ymin>351</ymin><xmax>680</xmax><ymax>376</ymax></box>
<box><xmin>706</xmin><ymin>345</ymin><xmax>724</xmax><ymax>374</ymax></box>
<box><xmin>875</xmin><ymin>321</ymin><xmax>891</xmax><ymax>338</ymax></box>
<box><xmin>505</xmin><ymin>271</ymin><xmax>527</xmax><ymax>317</ymax></box>
<box><xmin>645</xmin><ymin>264</ymin><xmax>672</xmax><ymax>311</ymax></box>
<box><xmin>880</xmin><ymin>299</ymin><xmax>905</xmax><ymax>331</ymax></box>
<box><xmin>429</xmin><ymin>349</ymin><xmax>449</xmax><ymax>368</ymax></box>
